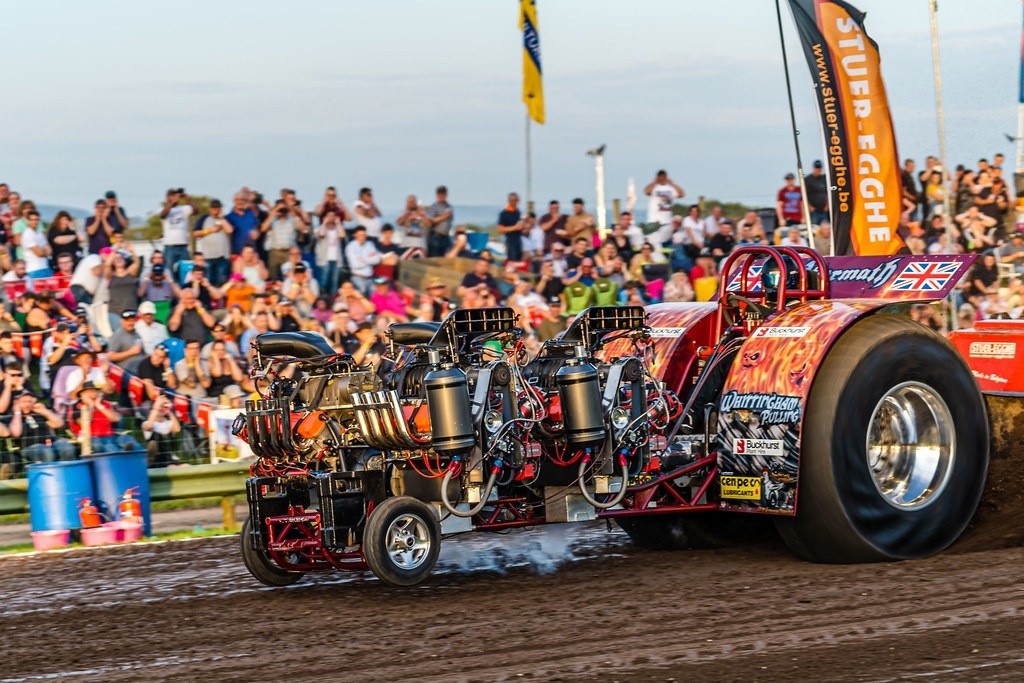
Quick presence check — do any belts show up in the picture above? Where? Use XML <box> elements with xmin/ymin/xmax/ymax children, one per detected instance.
<box><xmin>431</xmin><ymin>230</ymin><xmax>447</xmax><ymax>237</ymax></box>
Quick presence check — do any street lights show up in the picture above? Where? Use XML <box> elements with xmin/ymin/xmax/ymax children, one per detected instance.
<box><xmin>587</xmin><ymin>143</ymin><xmax>607</xmax><ymax>241</ymax></box>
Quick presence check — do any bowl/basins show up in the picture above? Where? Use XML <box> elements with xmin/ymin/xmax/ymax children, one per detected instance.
<box><xmin>102</xmin><ymin>520</ymin><xmax>143</xmax><ymax>541</ymax></box>
<box><xmin>79</xmin><ymin>527</ymin><xmax>123</xmax><ymax>544</ymax></box>
<box><xmin>30</xmin><ymin>528</ymin><xmax>71</xmax><ymax>548</ymax></box>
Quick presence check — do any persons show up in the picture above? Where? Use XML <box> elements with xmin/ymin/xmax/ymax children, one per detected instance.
<box><xmin>0</xmin><ymin>153</ymin><xmax>1024</xmax><ymax>467</ymax></box>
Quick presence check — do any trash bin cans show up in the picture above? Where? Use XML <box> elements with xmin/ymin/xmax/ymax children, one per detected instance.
<box><xmin>26</xmin><ymin>450</ymin><xmax>150</xmax><ymax>539</ymax></box>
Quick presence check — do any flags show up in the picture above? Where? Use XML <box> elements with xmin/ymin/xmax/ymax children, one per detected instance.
<box><xmin>518</xmin><ymin>0</ymin><xmax>545</xmax><ymax>128</ymax></box>
<box><xmin>789</xmin><ymin>0</ymin><xmax>910</xmax><ymax>257</ymax></box>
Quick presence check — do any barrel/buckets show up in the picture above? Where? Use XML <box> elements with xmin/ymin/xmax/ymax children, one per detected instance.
<box><xmin>79</xmin><ymin>449</ymin><xmax>151</xmax><ymax>537</ymax></box>
<box><xmin>26</xmin><ymin>460</ymin><xmax>95</xmax><ymax>543</ymax></box>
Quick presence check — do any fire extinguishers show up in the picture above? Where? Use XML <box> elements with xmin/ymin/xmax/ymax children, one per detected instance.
<box><xmin>119</xmin><ymin>486</ymin><xmax>145</xmax><ymax>538</ymax></box>
<box><xmin>77</xmin><ymin>497</ymin><xmax>101</xmax><ymax>527</ymax></box>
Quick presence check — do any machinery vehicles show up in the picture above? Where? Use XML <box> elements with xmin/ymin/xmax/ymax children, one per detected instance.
<box><xmin>232</xmin><ymin>239</ymin><xmax>1024</xmax><ymax>592</ymax></box>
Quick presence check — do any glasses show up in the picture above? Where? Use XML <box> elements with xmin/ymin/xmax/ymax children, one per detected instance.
<box><xmin>11</xmin><ymin>373</ymin><xmax>23</xmax><ymax>377</ymax></box>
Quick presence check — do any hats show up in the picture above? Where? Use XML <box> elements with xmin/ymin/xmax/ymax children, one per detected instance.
<box><xmin>57</xmin><ymin>324</ymin><xmax>67</xmax><ymax>331</ymax></box>
<box><xmin>152</xmin><ymin>263</ymin><xmax>164</xmax><ymax>276</ymax></box>
<box><xmin>361</xmin><ymin>188</ymin><xmax>370</xmax><ymax>193</ymax></box>
<box><xmin>294</xmin><ymin>262</ymin><xmax>306</xmax><ymax>274</ymax></box>
<box><xmin>155</xmin><ymin>343</ymin><xmax>169</xmax><ymax>355</ymax></box>
<box><xmin>76</xmin><ymin>380</ymin><xmax>101</xmax><ymax>398</ymax></box>
<box><xmin>222</xmin><ymin>384</ymin><xmax>245</xmax><ymax>399</ymax></box>
<box><xmin>785</xmin><ymin>173</ymin><xmax>794</xmax><ymax>179</ymax></box>
<box><xmin>230</xmin><ymin>272</ymin><xmax>246</xmax><ymax>282</ymax></box>
<box><xmin>99</xmin><ymin>247</ymin><xmax>112</xmax><ymax>254</ymax></box>
<box><xmin>212</xmin><ymin>324</ymin><xmax>226</xmax><ymax>332</ymax></box>
<box><xmin>814</xmin><ymin>160</ymin><xmax>822</xmax><ymax>168</ymax></box>
<box><xmin>73</xmin><ymin>348</ymin><xmax>97</xmax><ymax>359</ymax></box>
<box><xmin>121</xmin><ymin>301</ymin><xmax>157</xmax><ymax>319</ymax></box>
<box><xmin>552</xmin><ymin>242</ymin><xmax>564</xmax><ymax>250</ymax></box>
<box><xmin>210</xmin><ymin>199</ymin><xmax>222</xmax><ymax>208</ymax></box>
<box><xmin>572</xmin><ymin>198</ymin><xmax>583</xmax><ymax>205</ymax></box>
